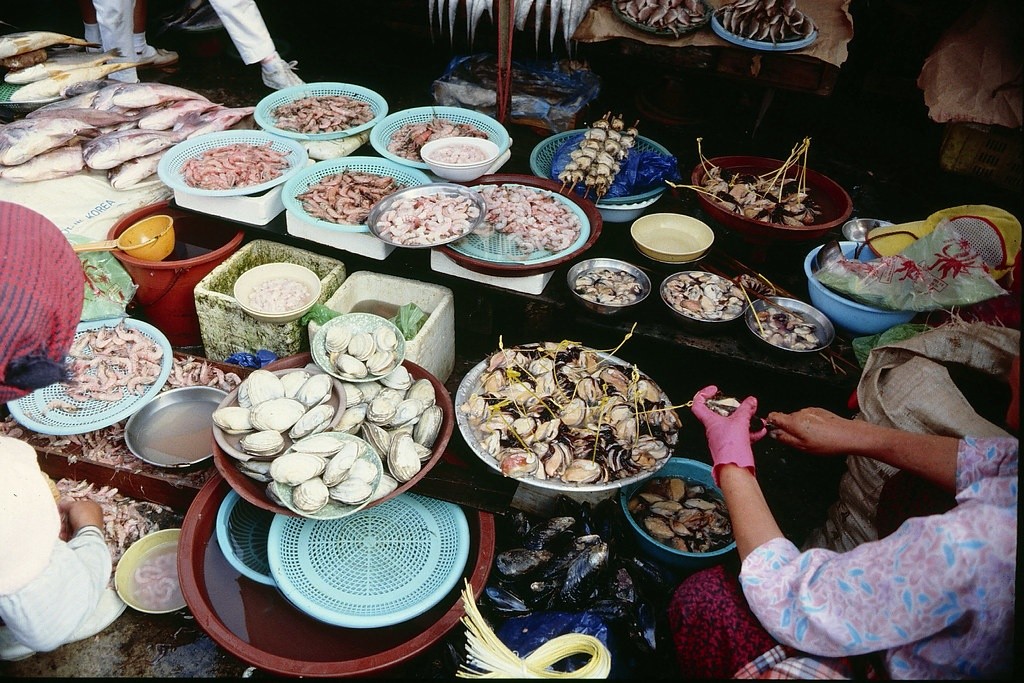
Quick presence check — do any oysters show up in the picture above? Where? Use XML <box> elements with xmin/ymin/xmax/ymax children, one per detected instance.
<box><xmin>635</xmin><ymin>479</ymin><xmax>731</xmax><ymax>550</ymax></box>
<box><xmin>212</xmin><ymin>323</ymin><xmax>443</xmax><ymax>511</ymax></box>
<box><xmin>472</xmin><ymin>344</ymin><xmax>681</xmax><ymax>480</ymax></box>
<box><xmin>666</xmin><ymin>272</ymin><xmax>742</xmax><ymax>320</ymax></box>
<box><xmin>753</xmin><ymin>310</ymin><xmax>818</xmax><ymax>351</ymax></box>
<box><xmin>575</xmin><ymin>270</ymin><xmax>641</xmax><ymax>304</ymax></box>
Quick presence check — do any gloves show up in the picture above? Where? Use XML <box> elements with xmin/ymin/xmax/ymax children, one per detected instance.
<box><xmin>691</xmin><ymin>385</ymin><xmax>768</xmax><ymax>490</ymax></box>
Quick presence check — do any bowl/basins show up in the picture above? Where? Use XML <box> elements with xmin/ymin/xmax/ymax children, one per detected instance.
<box><xmin>177</xmin><ymin>464</ymin><xmax>494</xmax><ymax>679</ymax></box>
<box><xmin>804</xmin><ymin>241</ymin><xmax>921</xmax><ymax>335</ymax></box>
<box><xmin>630</xmin><ymin>212</ymin><xmax>716</xmax><ymax>262</ymax></box>
<box><xmin>211</xmin><ymin>349</ymin><xmax>453</xmax><ymax>517</ymax></box>
<box><xmin>621</xmin><ymin>457</ymin><xmax>743</xmax><ymax>566</ymax></box>
<box><xmin>745</xmin><ymin>296</ymin><xmax>835</xmax><ymax>353</ymax></box>
<box><xmin>115</xmin><ymin>526</ymin><xmax>192</xmax><ymax>615</ymax></box>
<box><xmin>420</xmin><ymin>137</ymin><xmax>500</xmax><ymax>183</ymax></box>
<box><xmin>454</xmin><ymin>342</ymin><xmax>678</xmax><ymax>491</ymax></box>
<box><xmin>438</xmin><ymin>175</ymin><xmax>604</xmax><ymax>276</ymax></box>
<box><xmin>121</xmin><ymin>387</ymin><xmax>234</xmax><ymax>471</ymax></box>
<box><xmin>659</xmin><ymin>270</ymin><xmax>747</xmax><ymax>329</ymax></box>
<box><xmin>842</xmin><ymin>218</ymin><xmax>897</xmax><ymax>246</ymax></box>
<box><xmin>691</xmin><ymin>156</ymin><xmax>852</xmax><ymax>242</ymax></box>
<box><xmin>567</xmin><ymin>257</ymin><xmax>651</xmax><ymax>316</ymax></box>
<box><xmin>233</xmin><ymin>262</ymin><xmax>322</xmax><ymax>323</ymax></box>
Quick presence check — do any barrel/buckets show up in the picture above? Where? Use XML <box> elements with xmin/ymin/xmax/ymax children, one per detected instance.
<box><xmin>107</xmin><ymin>200</ymin><xmax>243</xmax><ymax>346</ymax></box>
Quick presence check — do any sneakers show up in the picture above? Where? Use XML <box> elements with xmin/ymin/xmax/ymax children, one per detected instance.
<box><xmin>261</xmin><ymin>60</ymin><xmax>305</xmax><ymax>90</ymax></box>
<box><xmin>136</xmin><ymin>48</ymin><xmax>180</xmax><ymax>70</ymax></box>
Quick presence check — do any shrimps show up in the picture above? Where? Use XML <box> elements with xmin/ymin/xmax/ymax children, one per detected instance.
<box><xmin>387</xmin><ymin>120</ymin><xmax>488</xmax><ymax>162</ymax></box>
<box><xmin>378</xmin><ymin>191</ymin><xmax>478</xmax><ymax>245</ymax></box>
<box><xmin>43</xmin><ymin>320</ymin><xmax>161</xmax><ymax>412</ymax></box>
<box><xmin>295</xmin><ymin>170</ymin><xmax>404</xmax><ymax>227</ymax></box>
<box><xmin>272</xmin><ymin>95</ymin><xmax>373</xmax><ymax>133</ymax></box>
<box><xmin>163</xmin><ymin>356</ymin><xmax>241</xmax><ymax>392</ymax></box>
<box><xmin>55</xmin><ymin>478</ymin><xmax>160</xmax><ymax>573</ymax></box>
<box><xmin>476</xmin><ymin>185</ymin><xmax>580</xmax><ymax>253</ymax></box>
<box><xmin>183</xmin><ymin>143</ymin><xmax>288</xmax><ymax>189</ymax></box>
<box><xmin>135</xmin><ymin>552</ymin><xmax>180</xmax><ymax>606</ymax></box>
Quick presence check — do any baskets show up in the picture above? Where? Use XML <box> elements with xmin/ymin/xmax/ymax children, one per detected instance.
<box><xmin>7</xmin><ymin>317</ymin><xmax>173</xmax><ymax>436</ymax></box>
<box><xmin>157</xmin><ymin>129</ymin><xmax>306</xmax><ymax>196</ymax></box>
<box><xmin>370</xmin><ymin>106</ymin><xmax>510</xmax><ymax>169</ymax></box>
<box><xmin>216</xmin><ymin>487</ymin><xmax>278</xmax><ymax>586</ymax></box>
<box><xmin>267</xmin><ymin>493</ymin><xmax>470</xmax><ymax>628</ymax></box>
<box><xmin>254</xmin><ymin>82</ymin><xmax>388</xmax><ymax>140</ymax></box>
<box><xmin>529</xmin><ymin>128</ymin><xmax>673</xmax><ymax>204</ymax></box>
<box><xmin>281</xmin><ymin>156</ymin><xmax>433</xmax><ymax>233</ymax></box>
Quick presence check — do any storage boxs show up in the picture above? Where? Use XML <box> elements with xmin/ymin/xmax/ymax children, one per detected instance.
<box><xmin>937</xmin><ymin>121</ymin><xmax>1024</xmax><ymax>184</ymax></box>
<box><xmin>307</xmin><ymin>269</ymin><xmax>454</xmax><ymax>387</ymax></box>
<box><xmin>193</xmin><ymin>239</ymin><xmax>346</xmax><ymax>369</ymax></box>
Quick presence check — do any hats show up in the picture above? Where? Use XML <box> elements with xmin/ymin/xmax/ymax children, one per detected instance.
<box><xmin>865</xmin><ymin>205</ymin><xmax>1022</xmax><ymax>281</ymax></box>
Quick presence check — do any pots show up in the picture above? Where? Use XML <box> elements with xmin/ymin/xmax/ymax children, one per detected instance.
<box><xmin>67</xmin><ymin>214</ymin><xmax>176</xmax><ymax>261</ymax></box>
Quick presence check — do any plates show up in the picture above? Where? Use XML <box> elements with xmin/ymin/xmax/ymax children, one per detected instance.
<box><xmin>609</xmin><ymin>1</ymin><xmax>819</xmax><ymax>51</ymax></box>
<box><xmin>633</xmin><ymin>242</ymin><xmax>713</xmax><ymax>266</ymax></box>
<box><xmin>367</xmin><ymin>182</ymin><xmax>486</xmax><ymax>249</ymax></box>
<box><xmin>210</xmin><ymin>313</ymin><xmax>454</xmax><ymax>521</ymax></box>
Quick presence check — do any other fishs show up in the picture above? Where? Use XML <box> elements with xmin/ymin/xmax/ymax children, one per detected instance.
<box><xmin>0</xmin><ymin>80</ymin><xmax>256</xmax><ymax>189</ymax></box>
<box><xmin>3</xmin><ymin>47</ymin><xmax>126</xmax><ymax>84</ymax></box>
<box><xmin>0</xmin><ymin>30</ymin><xmax>103</xmax><ymax>59</ymax></box>
<box><xmin>9</xmin><ymin>59</ymin><xmax>150</xmax><ymax>101</ymax></box>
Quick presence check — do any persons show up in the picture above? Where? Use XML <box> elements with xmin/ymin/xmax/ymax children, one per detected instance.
<box><xmin>0</xmin><ymin>200</ymin><xmax>128</xmax><ymax>661</ymax></box>
<box><xmin>670</xmin><ymin>385</ymin><xmax>1018</xmax><ymax>680</ymax></box>
<box><xmin>84</xmin><ymin>0</ymin><xmax>178</xmax><ymax>68</ymax></box>
<box><xmin>93</xmin><ymin>0</ymin><xmax>309</xmax><ymax>96</ymax></box>
